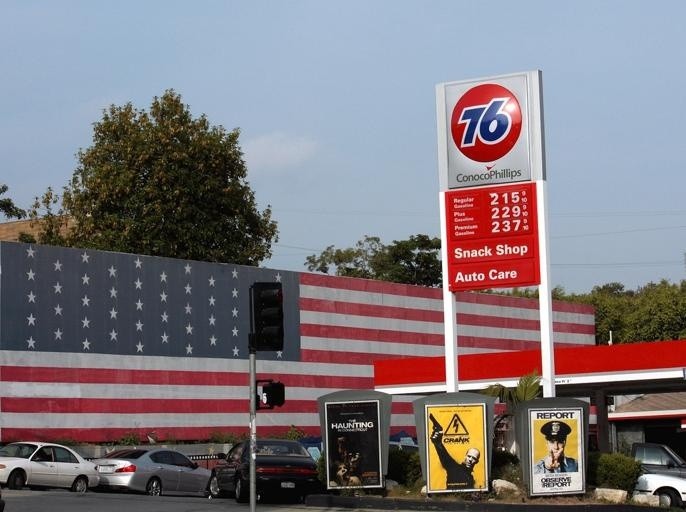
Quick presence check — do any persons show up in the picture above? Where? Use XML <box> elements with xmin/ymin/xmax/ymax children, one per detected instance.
<box><xmin>329</xmin><ymin>462</ymin><xmax>362</xmax><ymax>486</ymax></box>
<box><xmin>534</xmin><ymin>421</ymin><xmax>578</xmax><ymax>473</ymax></box>
<box><xmin>431</xmin><ymin>425</ymin><xmax>480</xmax><ymax>489</ymax></box>
<box><xmin>337</xmin><ymin>437</ymin><xmax>362</xmax><ymax>476</ymax></box>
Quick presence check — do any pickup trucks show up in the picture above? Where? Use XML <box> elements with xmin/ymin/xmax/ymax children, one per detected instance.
<box><xmin>604</xmin><ymin>442</ymin><xmax>685</xmax><ymax>507</ymax></box>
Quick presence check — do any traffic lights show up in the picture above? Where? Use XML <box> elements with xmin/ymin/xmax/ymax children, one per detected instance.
<box><xmin>251</xmin><ymin>282</ymin><xmax>283</xmax><ymax>350</ymax></box>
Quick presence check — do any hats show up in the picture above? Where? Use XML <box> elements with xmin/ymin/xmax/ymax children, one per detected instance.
<box><xmin>540</xmin><ymin>420</ymin><xmax>571</xmax><ymax>441</ymax></box>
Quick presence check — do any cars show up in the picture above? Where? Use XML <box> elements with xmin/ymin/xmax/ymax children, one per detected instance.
<box><xmin>2</xmin><ymin>443</ymin><xmax>101</xmax><ymax>495</ymax></box>
<box><xmin>209</xmin><ymin>440</ymin><xmax>320</xmax><ymax>503</ymax></box>
<box><xmin>89</xmin><ymin>448</ymin><xmax>209</xmax><ymax>496</ymax></box>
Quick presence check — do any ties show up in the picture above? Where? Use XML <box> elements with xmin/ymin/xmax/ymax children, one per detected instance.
<box><xmin>559</xmin><ymin>462</ymin><xmax>566</xmax><ymax>472</ymax></box>
